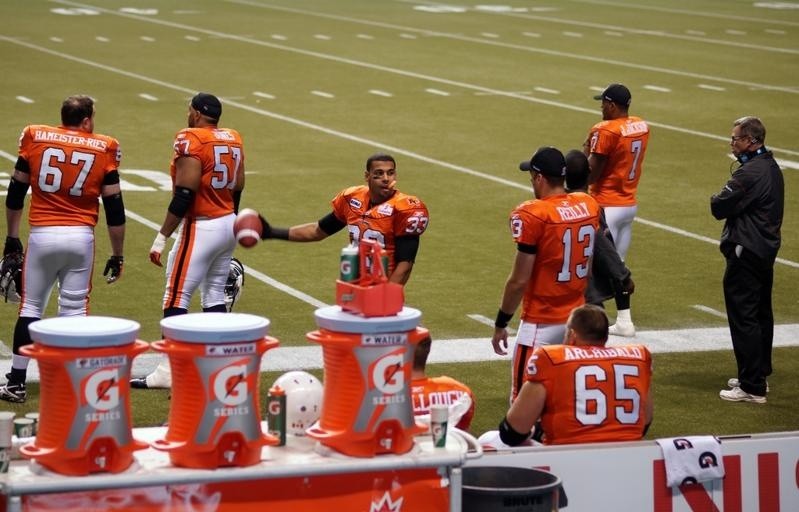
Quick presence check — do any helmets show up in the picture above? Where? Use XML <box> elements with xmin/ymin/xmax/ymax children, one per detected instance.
<box><xmin>224</xmin><ymin>257</ymin><xmax>245</xmax><ymax>313</ymax></box>
<box><xmin>0</xmin><ymin>252</ymin><xmax>25</xmax><ymax>304</ymax></box>
<box><xmin>270</xmin><ymin>370</ymin><xmax>325</xmax><ymax>435</ymax></box>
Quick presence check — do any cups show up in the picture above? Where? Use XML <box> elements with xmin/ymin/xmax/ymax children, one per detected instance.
<box><xmin>430</xmin><ymin>404</ymin><xmax>449</xmax><ymax>449</ymax></box>
<box><xmin>0</xmin><ymin>409</ymin><xmax>43</xmax><ymax>474</ymax></box>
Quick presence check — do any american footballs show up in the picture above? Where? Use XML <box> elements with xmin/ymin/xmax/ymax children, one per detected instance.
<box><xmin>233</xmin><ymin>207</ymin><xmax>263</xmax><ymax>247</ymax></box>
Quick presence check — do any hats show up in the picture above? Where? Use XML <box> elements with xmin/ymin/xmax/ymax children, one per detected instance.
<box><xmin>519</xmin><ymin>147</ymin><xmax>566</xmax><ymax>178</ymax></box>
<box><xmin>191</xmin><ymin>93</ymin><xmax>221</xmax><ymax>119</ymax></box>
<box><xmin>593</xmin><ymin>83</ymin><xmax>631</xmax><ymax>105</ymax></box>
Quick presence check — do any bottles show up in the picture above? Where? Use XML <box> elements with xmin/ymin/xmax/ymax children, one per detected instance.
<box><xmin>266</xmin><ymin>385</ymin><xmax>286</xmax><ymax>447</ymax></box>
<box><xmin>340</xmin><ymin>241</ymin><xmax>388</xmax><ymax>309</ymax></box>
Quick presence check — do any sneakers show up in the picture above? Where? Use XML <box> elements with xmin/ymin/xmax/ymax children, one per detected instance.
<box><xmin>608</xmin><ymin>323</ymin><xmax>635</xmax><ymax>336</ymax></box>
<box><xmin>130</xmin><ymin>355</ymin><xmax>172</xmax><ymax>388</ymax></box>
<box><xmin>0</xmin><ymin>373</ymin><xmax>27</xmax><ymax>403</ymax></box>
<box><xmin>727</xmin><ymin>378</ymin><xmax>769</xmax><ymax>392</ymax></box>
<box><xmin>719</xmin><ymin>387</ymin><xmax>768</xmax><ymax>404</ymax></box>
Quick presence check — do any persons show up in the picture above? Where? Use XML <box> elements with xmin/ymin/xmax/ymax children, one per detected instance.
<box><xmin>478</xmin><ymin>304</ymin><xmax>654</xmax><ymax>450</ymax></box>
<box><xmin>0</xmin><ymin>96</ymin><xmax>126</xmax><ymax>403</ymax></box>
<box><xmin>491</xmin><ymin>146</ymin><xmax>602</xmax><ymax>442</ymax></box>
<box><xmin>132</xmin><ymin>93</ymin><xmax>245</xmax><ymax>389</ymax></box>
<box><xmin>583</xmin><ymin>84</ymin><xmax>649</xmax><ymax>336</ymax></box>
<box><xmin>563</xmin><ymin>150</ymin><xmax>634</xmax><ymax>312</ymax></box>
<box><xmin>258</xmin><ymin>154</ymin><xmax>429</xmax><ymax>287</ymax></box>
<box><xmin>411</xmin><ymin>336</ymin><xmax>474</xmax><ymax>432</ymax></box>
<box><xmin>711</xmin><ymin>117</ymin><xmax>785</xmax><ymax>404</ymax></box>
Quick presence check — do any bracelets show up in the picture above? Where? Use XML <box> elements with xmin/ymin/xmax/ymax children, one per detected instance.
<box><xmin>271</xmin><ymin>228</ymin><xmax>289</xmax><ymax>240</ymax></box>
<box><xmin>495</xmin><ymin>309</ymin><xmax>514</xmax><ymax>329</ymax></box>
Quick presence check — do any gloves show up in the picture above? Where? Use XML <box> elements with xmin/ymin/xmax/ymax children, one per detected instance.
<box><xmin>4</xmin><ymin>235</ymin><xmax>23</xmax><ymax>254</ymax></box>
<box><xmin>150</xmin><ymin>231</ymin><xmax>167</xmax><ymax>267</ymax></box>
<box><xmin>258</xmin><ymin>214</ymin><xmax>272</xmax><ymax>240</ymax></box>
<box><xmin>104</xmin><ymin>255</ymin><xmax>124</xmax><ymax>283</ymax></box>
